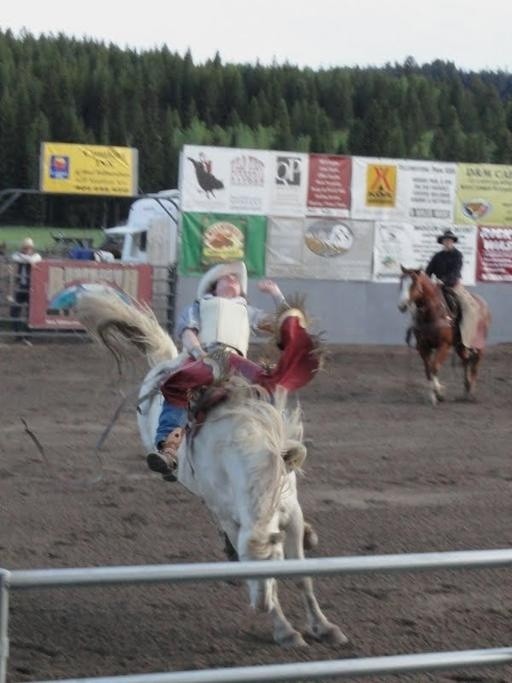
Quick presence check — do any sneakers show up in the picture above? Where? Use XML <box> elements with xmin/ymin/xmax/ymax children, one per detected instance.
<box><xmin>457</xmin><ymin>345</ymin><xmax>469</xmax><ymax>359</ymax></box>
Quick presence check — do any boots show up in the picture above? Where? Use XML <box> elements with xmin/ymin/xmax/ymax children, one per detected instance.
<box><xmin>283</xmin><ymin>446</ymin><xmax>306</xmax><ymax>474</ymax></box>
<box><xmin>147</xmin><ymin>428</ymin><xmax>183</xmax><ymax>481</ymax></box>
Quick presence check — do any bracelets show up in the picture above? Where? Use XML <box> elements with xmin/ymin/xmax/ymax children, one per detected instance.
<box><xmin>190</xmin><ymin>344</ymin><xmax>204</xmax><ymax>358</ymax></box>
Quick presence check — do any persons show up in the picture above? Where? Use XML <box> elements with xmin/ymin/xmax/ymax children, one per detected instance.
<box><xmin>9</xmin><ymin>235</ymin><xmax>42</xmax><ymax>346</ymax></box>
<box><xmin>148</xmin><ymin>260</ymin><xmax>287</xmax><ymax>483</ymax></box>
<box><xmin>101</xmin><ymin>237</ymin><xmax>122</xmax><ymax>259</ymax></box>
<box><xmin>424</xmin><ymin>226</ymin><xmax>471</xmax><ymax>360</ymax></box>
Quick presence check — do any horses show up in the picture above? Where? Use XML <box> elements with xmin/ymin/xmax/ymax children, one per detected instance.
<box><xmin>396</xmin><ymin>262</ymin><xmax>491</xmax><ymax>405</ymax></box>
<box><xmin>70</xmin><ymin>279</ymin><xmax>350</xmax><ymax>650</ymax></box>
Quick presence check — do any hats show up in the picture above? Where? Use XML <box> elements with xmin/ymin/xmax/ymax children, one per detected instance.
<box><xmin>438</xmin><ymin>231</ymin><xmax>457</xmax><ymax>244</ymax></box>
<box><xmin>197</xmin><ymin>262</ymin><xmax>248</xmax><ymax>298</ymax></box>
